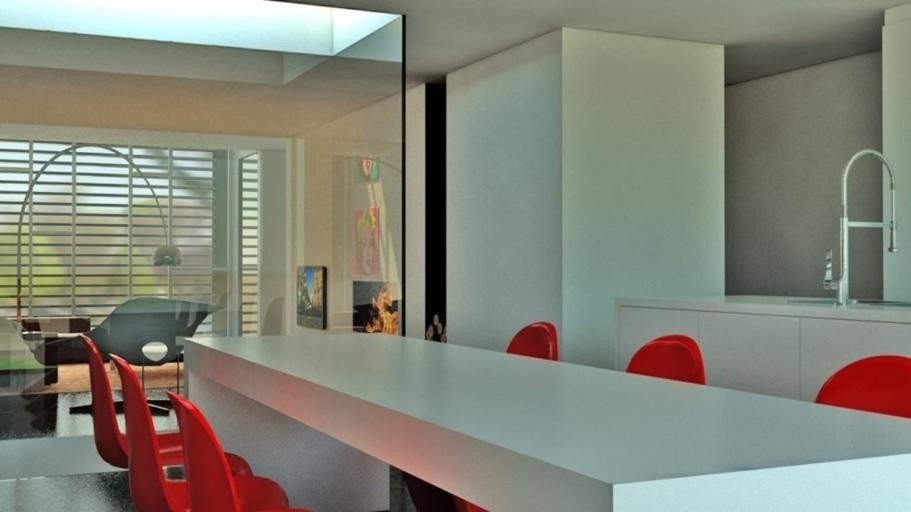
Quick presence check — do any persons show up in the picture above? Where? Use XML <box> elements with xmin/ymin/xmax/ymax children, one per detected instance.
<box><xmin>356</xmin><ymin>210</ymin><xmax>376</xmax><ymax>274</ymax></box>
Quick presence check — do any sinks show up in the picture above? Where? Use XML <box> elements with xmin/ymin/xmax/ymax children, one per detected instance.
<box><xmin>786</xmin><ymin>298</ymin><xmax>910</xmax><ymax>307</ymax></box>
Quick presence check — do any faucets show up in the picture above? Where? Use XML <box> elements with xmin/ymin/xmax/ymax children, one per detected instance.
<box><xmin>820</xmin><ymin>149</ymin><xmax>897</xmax><ymax>309</ymax></box>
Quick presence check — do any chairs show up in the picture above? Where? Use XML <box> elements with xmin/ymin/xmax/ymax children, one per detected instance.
<box><xmin>32</xmin><ymin>297</ymin><xmax>217</xmax><ymax>416</ymax></box>
<box><xmin>455</xmin><ymin>321</ymin><xmax>557</xmax><ymax>511</ymax></box>
<box><xmin>80</xmin><ymin>335</ymin><xmax>185</xmax><ymax>468</ymax></box>
<box><xmin>815</xmin><ymin>355</ymin><xmax>911</xmax><ymax>419</ymax></box>
<box><xmin>165</xmin><ymin>391</ymin><xmax>310</xmax><ymax>512</ymax></box>
<box><xmin>625</xmin><ymin>335</ymin><xmax>705</xmax><ymax>384</ymax></box>
<box><xmin>110</xmin><ymin>352</ymin><xmax>252</xmax><ymax>511</ymax></box>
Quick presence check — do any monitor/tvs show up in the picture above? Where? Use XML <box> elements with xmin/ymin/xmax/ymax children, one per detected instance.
<box><xmin>297</xmin><ymin>266</ymin><xmax>327</xmax><ymax>329</ymax></box>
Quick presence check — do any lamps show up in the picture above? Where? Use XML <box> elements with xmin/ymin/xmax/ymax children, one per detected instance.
<box><xmin>17</xmin><ymin>144</ymin><xmax>183</xmax><ymax>316</ymax></box>
